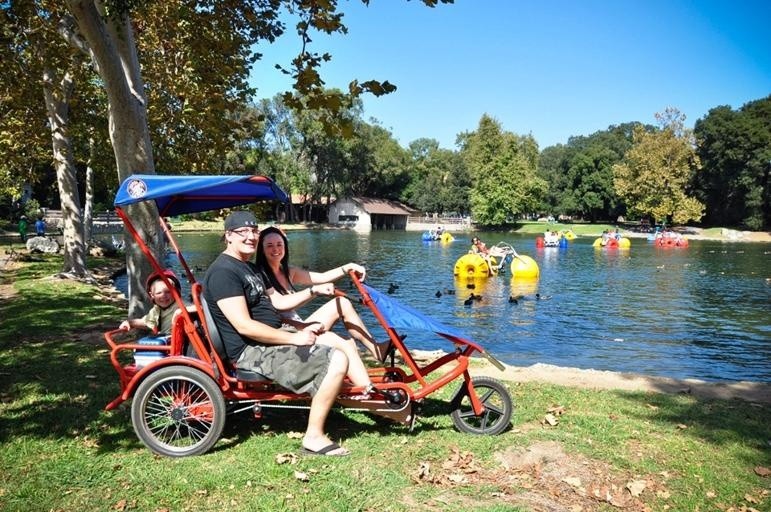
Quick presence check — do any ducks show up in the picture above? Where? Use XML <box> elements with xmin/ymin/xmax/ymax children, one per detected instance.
<box><xmin>508</xmin><ymin>294</ymin><xmax>525</xmax><ymax>304</ymax></box>
<box><xmin>464</xmin><ymin>292</ymin><xmax>483</xmax><ymax>306</ymax></box>
<box><xmin>435</xmin><ymin>287</ymin><xmax>456</xmax><ymax>297</ymax></box>
<box><xmin>534</xmin><ymin>293</ymin><xmax>552</xmax><ymax>300</ymax></box>
<box><xmin>387</xmin><ymin>282</ymin><xmax>400</xmax><ymax>294</ymax></box>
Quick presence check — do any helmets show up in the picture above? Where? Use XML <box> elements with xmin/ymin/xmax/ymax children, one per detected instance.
<box><xmin>145</xmin><ymin>269</ymin><xmax>181</xmax><ymax>299</ymax></box>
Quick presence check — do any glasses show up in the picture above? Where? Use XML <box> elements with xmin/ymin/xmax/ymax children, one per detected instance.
<box><xmin>230</xmin><ymin>229</ymin><xmax>261</xmax><ymax>237</ymax></box>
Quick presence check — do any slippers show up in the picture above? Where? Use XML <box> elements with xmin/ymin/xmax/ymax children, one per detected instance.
<box><xmin>381</xmin><ymin>335</ymin><xmax>406</xmax><ymax>364</ymax></box>
<box><xmin>298</xmin><ymin>443</ymin><xmax>351</xmax><ymax>456</ymax></box>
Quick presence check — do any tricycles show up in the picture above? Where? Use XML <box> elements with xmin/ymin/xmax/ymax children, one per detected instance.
<box><xmin>103</xmin><ymin>174</ymin><xmax>513</xmax><ymax>459</ymax></box>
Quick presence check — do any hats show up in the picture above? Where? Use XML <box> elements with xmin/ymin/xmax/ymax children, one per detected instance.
<box><xmin>219</xmin><ymin>211</ymin><xmax>257</xmax><ymax>242</ymax></box>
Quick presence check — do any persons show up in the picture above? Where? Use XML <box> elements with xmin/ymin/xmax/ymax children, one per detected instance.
<box><xmin>428</xmin><ymin>214</ymin><xmax>687</xmax><ymax>277</ymax></box>
<box><xmin>256</xmin><ymin>226</ymin><xmax>409</xmax><ymax>364</ymax></box>
<box><xmin>118</xmin><ymin>270</ymin><xmax>201</xmax><ymax>367</ymax></box>
<box><xmin>162</xmin><ymin>218</ymin><xmax>173</xmax><ymax>249</ymax></box>
<box><xmin>18</xmin><ymin>215</ymin><xmax>29</xmax><ymax>244</ymax></box>
<box><xmin>34</xmin><ymin>215</ymin><xmax>46</xmax><ymax>237</ymax></box>
<box><xmin>202</xmin><ymin>210</ymin><xmax>379</xmax><ymax>456</ymax></box>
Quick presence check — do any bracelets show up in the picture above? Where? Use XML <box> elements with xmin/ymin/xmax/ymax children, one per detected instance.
<box><xmin>340</xmin><ymin>265</ymin><xmax>347</xmax><ymax>275</ymax></box>
<box><xmin>309</xmin><ymin>286</ymin><xmax>314</xmax><ymax>297</ymax></box>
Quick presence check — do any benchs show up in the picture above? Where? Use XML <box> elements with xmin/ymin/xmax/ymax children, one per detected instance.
<box><xmin>106</xmin><ymin>314</ymin><xmax>185</xmax><ymax>393</ymax></box>
<box><xmin>190</xmin><ymin>281</ymin><xmax>273</xmax><ymax>386</ymax></box>
